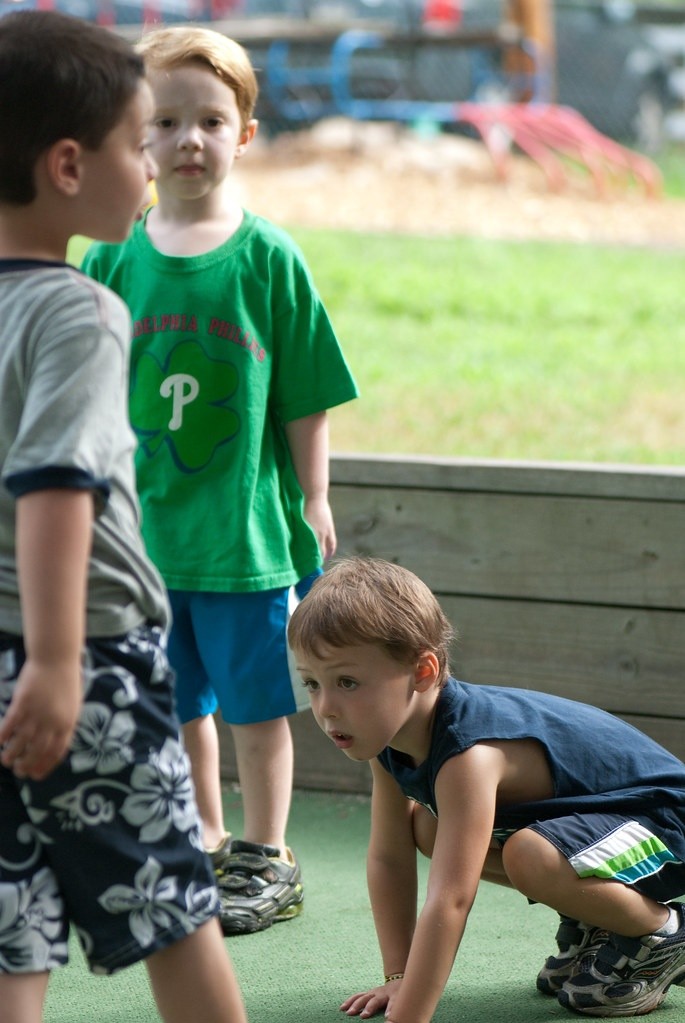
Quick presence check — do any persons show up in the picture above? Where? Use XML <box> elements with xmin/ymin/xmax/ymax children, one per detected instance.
<box><xmin>0</xmin><ymin>11</ymin><xmax>245</xmax><ymax>1023</ymax></box>
<box><xmin>288</xmin><ymin>557</ymin><xmax>685</xmax><ymax>1023</ymax></box>
<box><xmin>80</xmin><ymin>28</ymin><xmax>360</xmax><ymax>935</ymax></box>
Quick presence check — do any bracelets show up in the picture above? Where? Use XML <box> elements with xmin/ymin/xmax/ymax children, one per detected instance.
<box><xmin>385</xmin><ymin>976</ymin><xmax>403</xmax><ymax>983</ymax></box>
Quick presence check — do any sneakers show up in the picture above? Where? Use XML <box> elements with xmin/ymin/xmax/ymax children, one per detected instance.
<box><xmin>202</xmin><ymin>835</ymin><xmax>233</xmax><ymax>876</ymax></box>
<box><xmin>210</xmin><ymin>840</ymin><xmax>304</xmax><ymax>934</ymax></box>
<box><xmin>557</xmin><ymin>902</ymin><xmax>685</xmax><ymax>1017</ymax></box>
<box><xmin>536</xmin><ymin>913</ymin><xmax>601</xmax><ymax>995</ymax></box>
